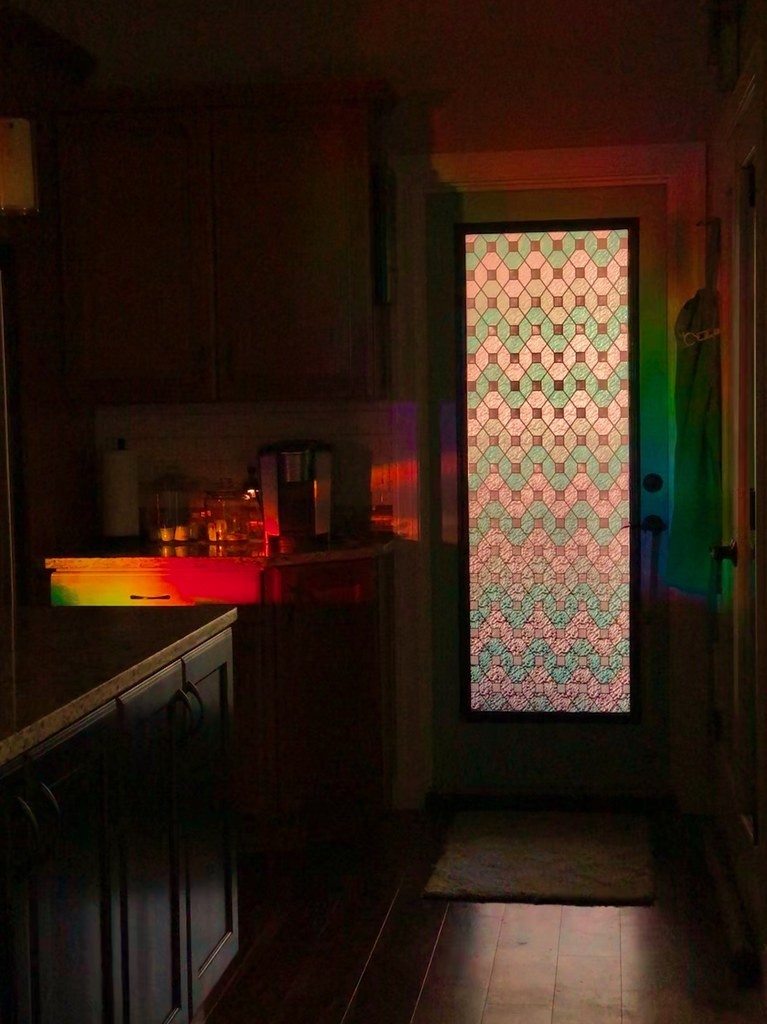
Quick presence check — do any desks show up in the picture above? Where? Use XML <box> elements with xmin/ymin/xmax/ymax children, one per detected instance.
<box><xmin>10</xmin><ymin>534</ymin><xmax>397</xmax><ymax>888</ymax></box>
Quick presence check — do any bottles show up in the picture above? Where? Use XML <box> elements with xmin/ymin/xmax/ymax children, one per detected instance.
<box><xmin>204</xmin><ymin>480</ymin><xmax>251</xmax><ymax>542</ymax></box>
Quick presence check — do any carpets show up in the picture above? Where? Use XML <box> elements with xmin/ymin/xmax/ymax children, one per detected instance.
<box><xmin>422</xmin><ymin>809</ymin><xmax>657</xmax><ymax>902</ymax></box>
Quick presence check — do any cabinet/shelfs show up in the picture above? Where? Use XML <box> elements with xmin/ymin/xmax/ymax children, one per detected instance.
<box><xmin>1</xmin><ymin>628</ymin><xmax>240</xmax><ymax>1024</ymax></box>
<box><xmin>51</xmin><ymin>82</ymin><xmax>397</xmax><ymax>415</ymax></box>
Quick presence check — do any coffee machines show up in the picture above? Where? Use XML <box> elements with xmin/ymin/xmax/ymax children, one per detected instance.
<box><xmin>255</xmin><ymin>442</ymin><xmax>331</xmax><ymax>545</ymax></box>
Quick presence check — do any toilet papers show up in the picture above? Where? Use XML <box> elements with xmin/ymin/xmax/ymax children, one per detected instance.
<box><xmin>103</xmin><ymin>447</ymin><xmax>140</xmax><ymax>537</ymax></box>
